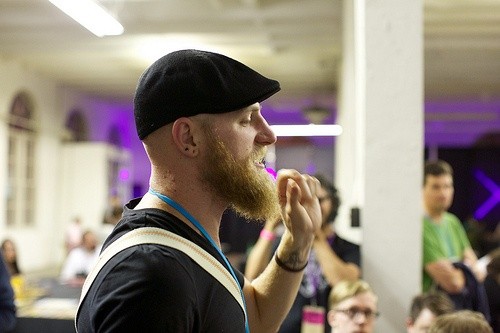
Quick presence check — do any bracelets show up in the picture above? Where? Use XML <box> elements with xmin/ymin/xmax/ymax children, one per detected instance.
<box><xmin>273</xmin><ymin>249</ymin><xmax>309</xmax><ymax>273</ymax></box>
<box><xmin>261</xmin><ymin>229</ymin><xmax>275</xmax><ymax>240</ymax></box>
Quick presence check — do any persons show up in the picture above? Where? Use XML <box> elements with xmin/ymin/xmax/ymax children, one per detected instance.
<box><xmin>221</xmin><ymin>160</ymin><xmax>500</xmax><ymax>333</ymax></box>
<box><xmin>0</xmin><ymin>204</ymin><xmax>124</xmax><ymax>333</ymax></box>
<box><xmin>75</xmin><ymin>49</ymin><xmax>322</xmax><ymax>333</ymax></box>
